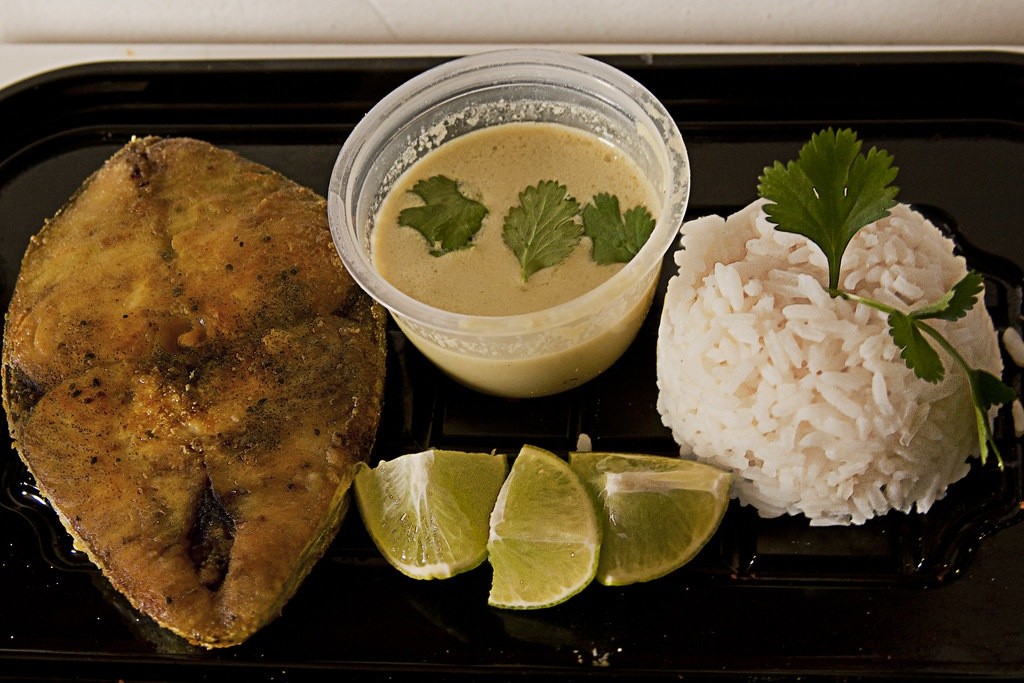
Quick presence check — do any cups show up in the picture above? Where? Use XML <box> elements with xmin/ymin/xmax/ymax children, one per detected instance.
<box><xmin>327</xmin><ymin>44</ymin><xmax>691</xmax><ymax>397</ymax></box>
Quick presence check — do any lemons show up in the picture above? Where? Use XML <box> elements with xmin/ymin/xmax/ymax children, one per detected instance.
<box><xmin>355</xmin><ymin>442</ymin><xmax>733</xmax><ymax>612</ymax></box>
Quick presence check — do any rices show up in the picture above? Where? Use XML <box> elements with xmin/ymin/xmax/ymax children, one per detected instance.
<box><xmin>653</xmin><ymin>123</ymin><xmax>1023</xmax><ymax>526</ymax></box>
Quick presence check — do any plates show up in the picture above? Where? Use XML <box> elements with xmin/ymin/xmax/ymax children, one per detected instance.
<box><xmin>0</xmin><ymin>50</ymin><xmax>1023</xmax><ymax>683</ymax></box>
